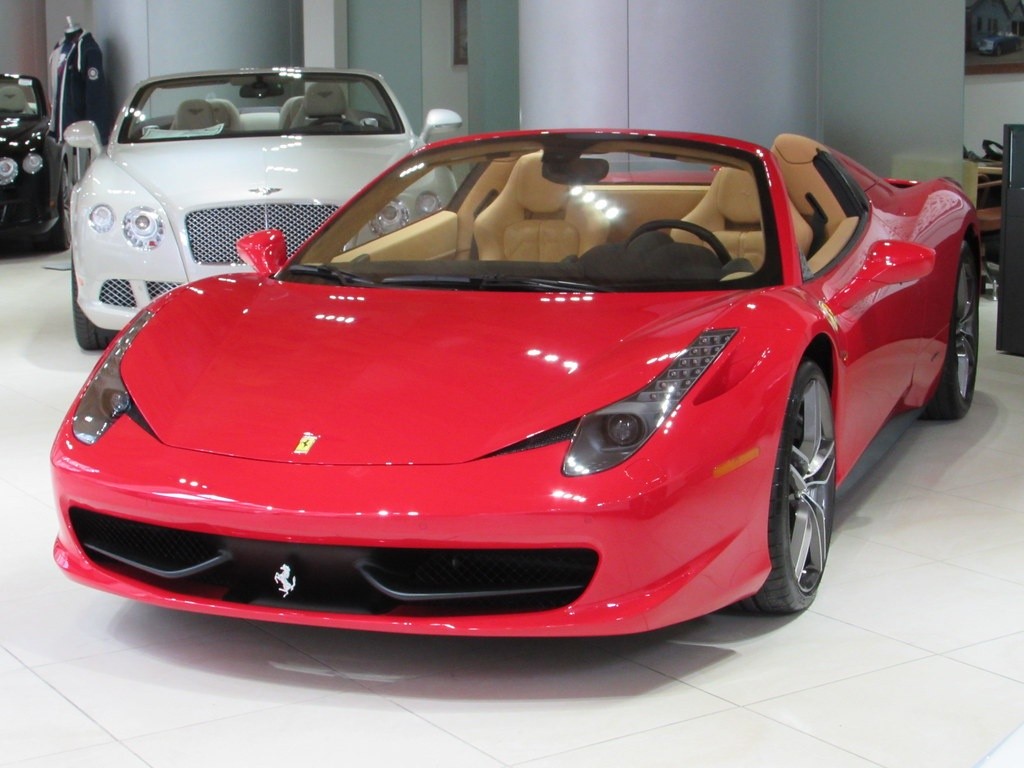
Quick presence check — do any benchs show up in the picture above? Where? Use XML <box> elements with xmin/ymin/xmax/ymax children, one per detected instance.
<box><xmin>171</xmin><ymin>96</ymin><xmax>304</xmax><ymax>130</ymax></box>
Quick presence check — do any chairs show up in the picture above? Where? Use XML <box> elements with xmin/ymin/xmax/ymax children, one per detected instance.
<box><xmin>473</xmin><ymin>152</ymin><xmax>612</xmax><ymax>263</ymax></box>
<box><xmin>177</xmin><ymin>99</ymin><xmax>214</xmax><ymax>129</ymax></box>
<box><xmin>290</xmin><ymin>83</ymin><xmax>346</xmax><ymax>128</ymax></box>
<box><xmin>0</xmin><ymin>85</ymin><xmax>33</xmax><ymax>115</ymax></box>
<box><xmin>669</xmin><ymin>166</ymin><xmax>814</xmax><ymax>271</ymax></box>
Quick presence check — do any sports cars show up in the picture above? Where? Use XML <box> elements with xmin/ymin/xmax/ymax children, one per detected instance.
<box><xmin>51</xmin><ymin>133</ymin><xmax>983</xmax><ymax>639</ymax></box>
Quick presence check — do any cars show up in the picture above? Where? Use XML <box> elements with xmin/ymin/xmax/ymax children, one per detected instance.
<box><xmin>0</xmin><ymin>74</ymin><xmax>74</xmax><ymax>251</ymax></box>
<box><xmin>976</xmin><ymin>30</ymin><xmax>1023</xmax><ymax>57</ymax></box>
<box><xmin>61</xmin><ymin>67</ymin><xmax>462</xmax><ymax>350</ymax></box>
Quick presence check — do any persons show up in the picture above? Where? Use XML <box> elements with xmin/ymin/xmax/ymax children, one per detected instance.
<box><xmin>46</xmin><ymin>27</ymin><xmax>109</xmax><ymax>147</ymax></box>
